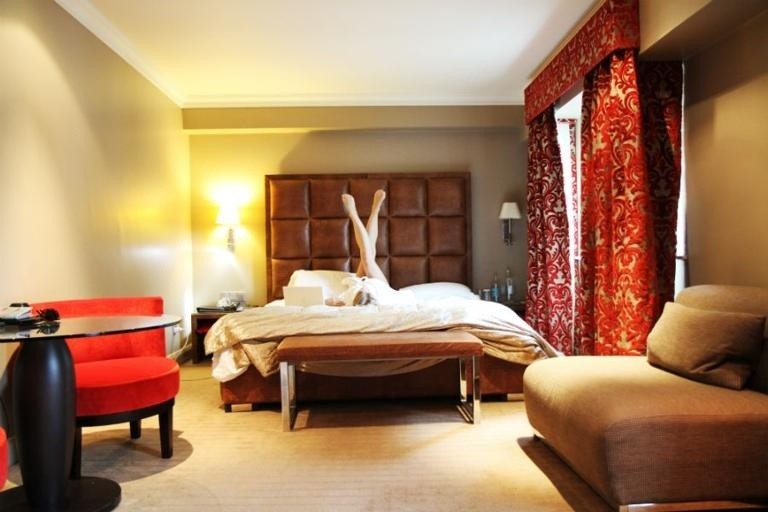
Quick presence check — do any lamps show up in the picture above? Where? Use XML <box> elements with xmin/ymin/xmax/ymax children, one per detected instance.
<box><xmin>497</xmin><ymin>198</ymin><xmax>524</xmax><ymax>247</ymax></box>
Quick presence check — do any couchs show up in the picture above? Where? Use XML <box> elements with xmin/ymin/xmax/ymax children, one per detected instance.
<box><xmin>22</xmin><ymin>291</ymin><xmax>182</xmax><ymax>460</ymax></box>
<box><xmin>520</xmin><ymin>275</ymin><xmax>768</xmax><ymax>512</ymax></box>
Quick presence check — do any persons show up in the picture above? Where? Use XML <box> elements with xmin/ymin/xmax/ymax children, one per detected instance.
<box><xmin>321</xmin><ymin>188</ymin><xmax>399</xmax><ymax>307</ymax></box>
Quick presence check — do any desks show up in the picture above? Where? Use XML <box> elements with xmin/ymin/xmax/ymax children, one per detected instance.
<box><xmin>0</xmin><ymin>314</ymin><xmax>183</xmax><ymax>512</ymax></box>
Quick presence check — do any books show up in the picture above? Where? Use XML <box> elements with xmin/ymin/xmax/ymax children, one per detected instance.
<box><xmin>196</xmin><ymin>306</ymin><xmax>224</xmax><ymax>313</ymax></box>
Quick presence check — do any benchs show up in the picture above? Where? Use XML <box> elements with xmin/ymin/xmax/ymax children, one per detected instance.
<box><xmin>271</xmin><ymin>327</ymin><xmax>486</xmax><ymax>435</ymax></box>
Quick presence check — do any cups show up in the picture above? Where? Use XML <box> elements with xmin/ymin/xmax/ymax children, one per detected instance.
<box><xmin>478</xmin><ymin>288</ymin><xmax>491</xmax><ymax>301</ymax></box>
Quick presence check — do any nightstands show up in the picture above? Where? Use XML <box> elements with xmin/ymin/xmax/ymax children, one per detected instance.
<box><xmin>491</xmin><ymin>295</ymin><xmax>527</xmax><ymax>322</ymax></box>
<box><xmin>188</xmin><ymin>307</ymin><xmax>244</xmax><ymax>367</ymax></box>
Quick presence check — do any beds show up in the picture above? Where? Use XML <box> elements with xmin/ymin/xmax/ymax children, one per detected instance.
<box><xmin>201</xmin><ymin>172</ymin><xmax>543</xmax><ymax>415</ymax></box>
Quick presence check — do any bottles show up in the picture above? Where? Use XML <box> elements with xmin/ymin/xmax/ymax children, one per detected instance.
<box><xmin>503</xmin><ymin>268</ymin><xmax>515</xmax><ymax>301</ymax></box>
<box><xmin>490</xmin><ymin>272</ymin><xmax>502</xmax><ymax>301</ymax></box>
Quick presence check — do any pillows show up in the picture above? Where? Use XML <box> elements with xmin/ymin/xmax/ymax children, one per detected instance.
<box><xmin>397</xmin><ymin>281</ymin><xmax>482</xmax><ymax>302</ymax></box>
<box><xmin>643</xmin><ymin>300</ymin><xmax>767</xmax><ymax>391</ymax></box>
<box><xmin>286</xmin><ymin>268</ymin><xmax>357</xmax><ymax>300</ymax></box>
